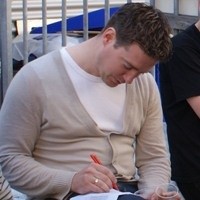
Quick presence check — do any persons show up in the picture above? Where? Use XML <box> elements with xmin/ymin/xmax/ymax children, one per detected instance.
<box><xmin>0</xmin><ymin>2</ymin><xmax>182</xmax><ymax>200</ymax></box>
<box><xmin>162</xmin><ymin>18</ymin><xmax>200</xmax><ymax>199</ymax></box>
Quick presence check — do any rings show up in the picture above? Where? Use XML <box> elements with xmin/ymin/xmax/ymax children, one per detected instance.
<box><xmin>94</xmin><ymin>178</ymin><xmax>98</xmax><ymax>185</ymax></box>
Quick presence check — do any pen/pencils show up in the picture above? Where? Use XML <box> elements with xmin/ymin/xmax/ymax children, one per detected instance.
<box><xmin>90</xmin><ymin>153</ymin><xmax>120</xmax><ymax>192</ymax></box>
